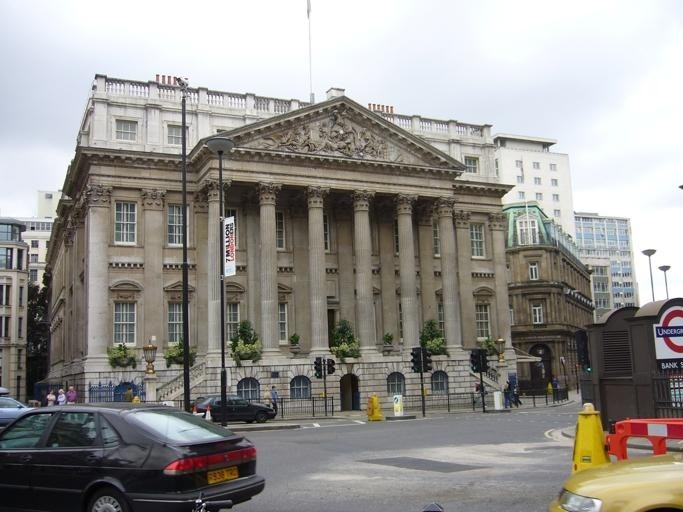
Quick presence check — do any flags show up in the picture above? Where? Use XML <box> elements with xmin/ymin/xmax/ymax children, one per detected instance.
<box><xmin>221</xmin><ymin>215</ymin><xmax>237</xmax><ymax>277</ymax></box>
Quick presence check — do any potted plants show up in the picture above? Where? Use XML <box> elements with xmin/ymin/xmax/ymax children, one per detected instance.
<box><xmin>382</xmin><ymin>332</ymin><xmax>395</xmax><ymax>352</ymax></box>
<box><xmin>329</xmin><ymin>320</ymin><xmax>362</xmax><ymax>359</ymax></box>
<box><xmin>226</xmin><ymin>319</ymin><xmax>264</xmax><ymax>366</ymax></box>
<box><xmin>482</xmin><ymin>338</ymin><xmax>497</xmax><ymax>357</ymax></box>
<box><xmin>162</xmin><ymin>337</ymin><xmax>197</xmax><ymax>368</ymax></box>
<box><xmin>288</xmin><ymin>332</ymin><xmax>302</xmax><ymax>353</ymax></box>
<box><xmin>418</xmin><ymin>319</ymin><xmax>450</xmax><ymax>359</ymax></box>
<box><xmin>108</xmin><ymin>342</ymin><xmax>136</xmax><ymax>371</ymax></box>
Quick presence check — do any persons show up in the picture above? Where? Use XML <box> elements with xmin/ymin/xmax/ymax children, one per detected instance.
<box><xmin>45</xmin><ymin>388</ymin><xmax>55</xmax><ymax>406</ymax></box>
<box><xmin>65</xmin><ymin>385</ymin><xmax>76</xmax><ymax>404</ymax></box>
<box><xmin>124</xmin><ymin>387</ymin><xmax>134</xmax><ymax>402</ymax></box>
<box><xmin>474</xmin><ymin>382</ymin><xmax>488</xmax><ymax>409</ymax></box>
<box><xmin>271</xmin><ymin>386</ymin><xmax>278</xmax><ymax>415</ymax></box>
<box><xmin>56</xmin><ymin>388</ymin><xmax>65</xmax><ymax>405</ymax></box>
<box><xmin>502</xmin><ymin>377</ymin><xmax>523</xmax><ymax>408</ymax></box>
<box><xmin>263</xmin><ymin>387</ymin><xmax>271</xmax><ymax>408</ymax></box>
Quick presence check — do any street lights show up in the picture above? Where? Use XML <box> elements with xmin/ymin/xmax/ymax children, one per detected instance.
<box><xmin>205</xmin><ymin>138</ymin><xmax>237</xmax><ymax>426</ymax></box>
<box><xmin>641</xmin><ymin>248</ymin><xmax>657</xmax><ymax>302</ymax></box>
<box><xmin>658</xmin><ymin>265</ymin><xmax>671</xmax><ymax>299</ymax></box>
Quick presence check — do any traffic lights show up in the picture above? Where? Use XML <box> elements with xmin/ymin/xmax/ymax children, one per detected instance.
<box><xmin>327</xmin><ymin>358</ymin><xmax>335</xmax><ymax>374</ymax></box>
<box><xmin>423</xmin><ymin>353</ymin><xmax>432</xmax><ymax>372</ymax></box>
<box><xmin>411</xmin><ymin>347</ymin><xmax>422</xmax><ymax>373</ymax></box>
<box><xmin>314</xmin><ymin>357</ymin><xmax>322</xmax><ymax>379</ymax></box>
<box><xmin>471</xmin><ymin>349</ymin><xmax>489</xmax><ymax>373</ymax></box>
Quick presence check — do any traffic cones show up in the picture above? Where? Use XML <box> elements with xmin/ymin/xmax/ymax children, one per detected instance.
<box><xmin>205</xmin><ymin>405</ymin><xmax>213</xmax><ymax>422</ymax></box>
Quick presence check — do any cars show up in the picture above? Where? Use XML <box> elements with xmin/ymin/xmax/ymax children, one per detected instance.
<box><xmin>0</xmin><ymin>397</ymin><xmax>40</xmax><ymax>427</ymax></box>
<box><xmin>547</xmin><ymin>452</ymin><xmax>683</xmax><ymax>512</ymax></box>
<box><xmin>191</xmin><ymin>395</ymin><xmax>277</xmax><ymax>422</ymax></box>
<box><xmin>0</xmin><ymin>403</ymin><xmax>265</xmax><ymax>512</ymax></box>
